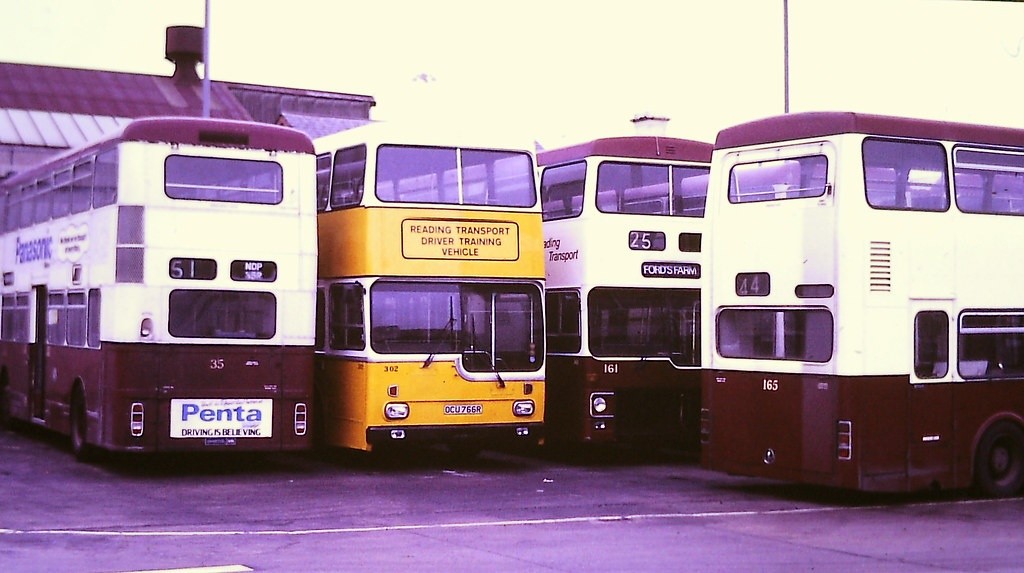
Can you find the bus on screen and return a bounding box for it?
[0,116,318,465]
[699,111,1023,500]
[175,127,546,468]
[333,136,1024,461]
